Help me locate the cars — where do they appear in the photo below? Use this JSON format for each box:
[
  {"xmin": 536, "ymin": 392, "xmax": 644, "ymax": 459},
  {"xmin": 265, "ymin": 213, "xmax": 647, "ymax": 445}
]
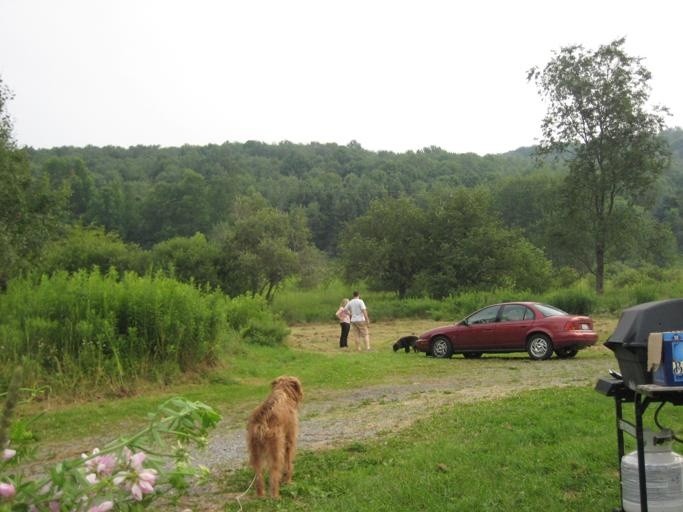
[{"xmin": 415, "ymin": 301, "xmax": 600, "ymax": 360}]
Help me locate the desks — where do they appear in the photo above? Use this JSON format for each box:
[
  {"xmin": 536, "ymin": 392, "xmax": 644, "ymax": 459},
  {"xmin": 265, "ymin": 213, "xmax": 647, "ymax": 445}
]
[{"xmin": 597, "ymin": 381, "xmax": 683, "ymax": 512}]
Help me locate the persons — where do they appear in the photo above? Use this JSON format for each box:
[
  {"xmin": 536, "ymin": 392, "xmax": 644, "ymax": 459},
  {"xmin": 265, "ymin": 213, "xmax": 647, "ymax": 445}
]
[
  {"xmin": 343, "ymin": 290, "xmax": 370, "ymax": 352},
  {"xmin": 335, "ymin": 298, "xmax": 351, "ymax": 348}
]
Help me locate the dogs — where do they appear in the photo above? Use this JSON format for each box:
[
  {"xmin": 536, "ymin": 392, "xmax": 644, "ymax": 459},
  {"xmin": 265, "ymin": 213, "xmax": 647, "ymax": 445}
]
[
  {"xmin": 246, "ymin": 375, "xmax": 304, "ymax": 501},
  {"xmin": 393, "ymin": 335, "xmax": 418, "ymax": 353}
]
